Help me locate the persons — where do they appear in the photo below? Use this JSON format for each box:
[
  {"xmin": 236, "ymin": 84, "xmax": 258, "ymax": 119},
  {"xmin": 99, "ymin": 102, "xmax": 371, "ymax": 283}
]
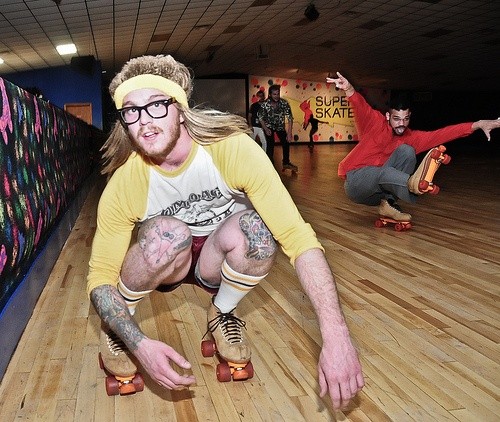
[
  {"xmin": 326, "ymin": 72, "xmax": 500, "ymax": 231},
  {"xmin": 85, "ymin": 55, "xmax": 365, "ymax": 411},
  {"xmin": 248, "ymin": 91, "xmax": 267, "ymax": 152},
  {"xmin": 258, "ymin": 85, "xmax": 298, "ymax": 173},
  {"xmin": 305, "ymin": 114, "xmax": 328, "ymax": 148}
]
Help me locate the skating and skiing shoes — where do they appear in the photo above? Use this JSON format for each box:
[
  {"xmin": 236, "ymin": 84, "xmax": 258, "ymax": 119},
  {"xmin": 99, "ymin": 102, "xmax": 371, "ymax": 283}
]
[
  {"xmin": 375, "ymin": 199, "xmax": 412, "ymax": 231},
  {"xmin": 201, "ymin": 295, "xmax": 253, "ymax": 382},
  {"xmin": 99, "ymin": 315, "xmax": 144, "ymax": 396},
  {"xmin": 408, "ymin": 145, "xmax": 451, "ymax": 196},
  {"xmin": 282, "ymin": 163, "xmax": 298, "ymax": 174}
]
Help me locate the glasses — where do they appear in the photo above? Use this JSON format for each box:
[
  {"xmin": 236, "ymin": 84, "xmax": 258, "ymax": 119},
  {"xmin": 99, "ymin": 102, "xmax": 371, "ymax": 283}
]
[{"xmin": 116, "ymin": 97, "xmax": 176, "ymax": 124}]
